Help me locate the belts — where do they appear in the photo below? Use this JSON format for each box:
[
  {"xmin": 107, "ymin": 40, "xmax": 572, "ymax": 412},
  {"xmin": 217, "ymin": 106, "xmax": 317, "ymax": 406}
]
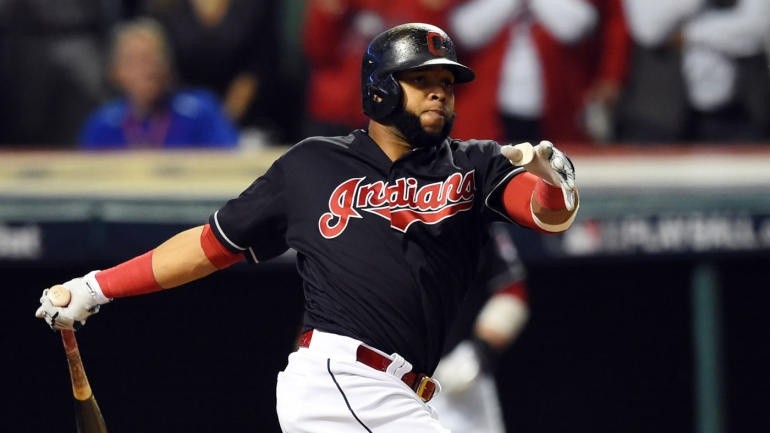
[{"xmin": 299, "ymin": 329, "xmax": 435, "ymax": 405}]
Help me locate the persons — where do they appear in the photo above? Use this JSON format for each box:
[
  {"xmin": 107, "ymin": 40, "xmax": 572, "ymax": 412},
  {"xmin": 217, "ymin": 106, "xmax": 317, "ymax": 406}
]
[
  {"xmin": 35, "ymin": 23, "xmax": 579, "ymax": 433},
  {"xmin": 69, "ymin": 16, "xmax": 238, "ymax": 149},
  {"xmin": 431, "ymin": 222, "xmax": 530, "ymax": 433},
  {"xmin": 302, "ymin": 0, "xmax": 770, "ymax": 145},
  {"xmin": 169, "ymin": 0, "xmax": 265, "ymax": 124}
]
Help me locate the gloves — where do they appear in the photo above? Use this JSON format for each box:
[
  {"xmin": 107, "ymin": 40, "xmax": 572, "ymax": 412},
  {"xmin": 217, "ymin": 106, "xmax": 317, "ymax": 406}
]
[
  {"xmin": 501, "ymin": 139, "xmax": 577, "ymax": 211},
  {"xmin": 34, "ymin": 270, "xmax": 112, "ymax": 332}
]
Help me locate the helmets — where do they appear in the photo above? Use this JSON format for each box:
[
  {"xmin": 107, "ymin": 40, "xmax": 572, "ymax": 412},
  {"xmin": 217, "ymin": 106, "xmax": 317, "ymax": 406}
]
[{"xmin": 362, "ymin": 22, "xmax": 477, "ymax": 124}]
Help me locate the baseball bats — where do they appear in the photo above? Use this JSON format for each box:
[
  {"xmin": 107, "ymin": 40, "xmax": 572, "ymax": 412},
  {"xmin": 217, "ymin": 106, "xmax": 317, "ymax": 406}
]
[{"xmin": 48, "ymin": 284, "xmax": 108, "ymax": 433}]
